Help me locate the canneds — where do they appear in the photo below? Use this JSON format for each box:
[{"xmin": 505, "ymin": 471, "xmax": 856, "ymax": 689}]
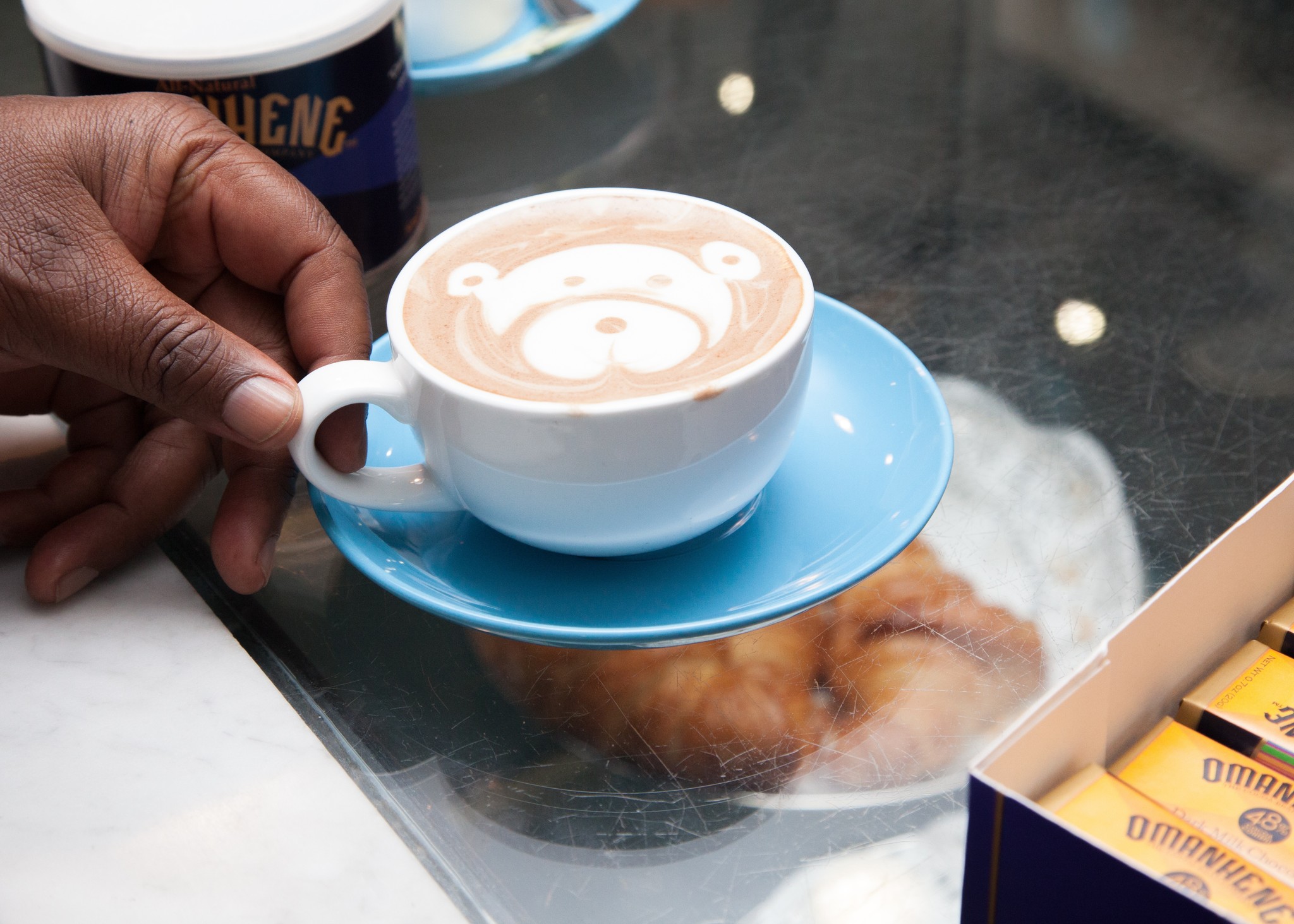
[{"xmin": 21, "ymin": 0, "xmax": 426, "ymax": 308}]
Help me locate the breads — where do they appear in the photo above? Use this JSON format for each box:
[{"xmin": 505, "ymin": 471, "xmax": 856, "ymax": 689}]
[{"xmin": 463, "ymin": 535, "xmax": 1052, "ymax": 790}]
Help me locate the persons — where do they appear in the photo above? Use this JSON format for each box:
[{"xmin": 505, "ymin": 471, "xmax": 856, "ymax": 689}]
[{"xmin": 1, "ymin": 89, "xmax": 370, "ymax": 608}]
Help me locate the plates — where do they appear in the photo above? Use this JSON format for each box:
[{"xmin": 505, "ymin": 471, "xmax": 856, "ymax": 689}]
[
  {"xmin": 382, "ymin": 1, "xmax": 643, "ymax": 91},
  {"xmin": 312, "ymin": 276, "xmax": 956, "ymax": 650},
  {"xmin": 657, "ymin": 378, "xmax": 1150, "ymax": 817}
]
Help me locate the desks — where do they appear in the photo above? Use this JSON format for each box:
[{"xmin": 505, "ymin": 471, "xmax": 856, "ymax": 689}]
[{"xmin": 5, "ymin": 1, "xmax": 1293, "ymax": 924}]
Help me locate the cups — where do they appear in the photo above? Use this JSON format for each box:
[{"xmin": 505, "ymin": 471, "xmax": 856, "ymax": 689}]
[
  {"xmin": 24, "ymin": 1, "xmax": 428, "ymax": 291},
  {"xmin": 283, "ymin": 183, "xmax": 813, "ymax": 562}
]
